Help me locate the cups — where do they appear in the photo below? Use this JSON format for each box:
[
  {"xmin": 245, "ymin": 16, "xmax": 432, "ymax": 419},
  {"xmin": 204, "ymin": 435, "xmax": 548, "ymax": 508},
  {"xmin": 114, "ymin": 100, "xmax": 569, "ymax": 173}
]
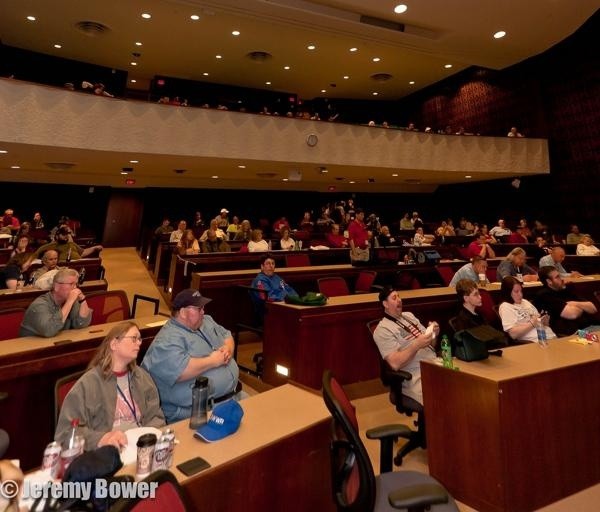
[
  {"xmin": 135, "ymin": 434, "xmax": 157, "ymax": 480},
  {"xmin": 78, "ymin": 267, "xmax": 86, "ymax": 285},
  {"xmin": 479, "ymin": 274, "xmax": 486, "ymax": 287},
  {"xmin": 65, "ymin": 248, "xmax": 74, "ymax": 262}
]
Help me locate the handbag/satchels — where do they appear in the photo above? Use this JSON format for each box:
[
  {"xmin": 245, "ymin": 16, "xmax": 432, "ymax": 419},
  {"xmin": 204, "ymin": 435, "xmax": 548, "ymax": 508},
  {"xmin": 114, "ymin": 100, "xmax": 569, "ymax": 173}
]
[
  {"xmin": 284, "ymin": 292, "xmax": 327, "ymax": 305},
  {"xmin": 454, "ymin": 324, "xmax": 504, "ymax": 362}
]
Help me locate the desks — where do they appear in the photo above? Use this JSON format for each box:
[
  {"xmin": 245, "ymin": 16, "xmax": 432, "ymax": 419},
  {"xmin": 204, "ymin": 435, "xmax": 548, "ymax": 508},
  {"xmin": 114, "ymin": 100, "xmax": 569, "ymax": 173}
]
[
  {"xmin": 1, "ymin": 278, "xmax": 108, "ymax": 317},
  {"xmin": 164, "ymin": 242, "xmax": 600, "ymax": 301},
  {"xmin": 189, "ymin": 256, "xmax": 599, "ymax": 336},
  {"xmin": 420, "ymin": 330, "xmax": 600, "ymax": 511},
  {"xmin": 2, "ymin": 257, "xmax": 104, "ymax": 289},
  {"xmin": 0, "ymin": 235, "xmax": 95, "ymax": 250},
  {"xmin": 152, "ymin": 239, "xmax": 295, "ymax": 285},
  {"xmin": 16, "ymin": 380, "xmax": 357, "ymax": 511},
  {"xmin": 261, "ymin": 274, "xmax": 600, "ymax": 390},
  {"xmin": 1, "ymin": 314, "xmax": 175, "ymax": 443}
]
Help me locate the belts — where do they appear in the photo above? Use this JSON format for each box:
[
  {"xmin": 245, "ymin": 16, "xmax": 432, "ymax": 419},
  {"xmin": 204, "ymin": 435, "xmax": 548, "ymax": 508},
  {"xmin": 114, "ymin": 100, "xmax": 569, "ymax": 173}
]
[{"xmin": 208, "ymin": 381, "xmax": 242, "ymax": 408}]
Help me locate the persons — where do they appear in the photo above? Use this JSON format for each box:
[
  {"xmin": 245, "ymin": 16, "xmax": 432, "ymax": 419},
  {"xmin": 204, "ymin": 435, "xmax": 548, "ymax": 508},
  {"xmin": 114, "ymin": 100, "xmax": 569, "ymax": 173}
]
[
  {"xmin": 534, "ymin": 266, "xmax": 600, "ymax": 335},
  {"xmin": 19, "ymin": 269, "xmax": 95, "ymax": 378},
  {"xmin": 155, "ymin": 199, "xmax": 600, "ymax": 262},
  {"xmin": 0, "ymin": 208, "xmax": 106, "ymax": 290},
  {"xmin": 448, "ymin": 255, "xmax": 490, "ymax": 288},
  {"xmin": 250, "ymin": 254, "xmax": 299, "ymax": 304},
  {"xmin": 373, "ymin": 286, "xmax": 444, "ymax": 407},
  {"xmin": 538, "ymin": 247, "xmax": 583, "ymax": 278},
  {"xmin": 63, "ymin": 82, "xmax": 522, "ymax": 137},
  {"xmin": 497, "ymin": 276, "xmax": 558, "ymax": 343},
  {"xmin": 53, "ymin": 321, "xmax": 167, "ymax": 454},
  {"xmin": 496, "ymin": 247, "xmax": 539, "ymax": 281},
  {"xmin": 139, "ymin": 288, "xmax": 251, "ymax": 426},
  {"xmin": 454, "ymin": 278, "xmax": 506, "ymax": 350}
]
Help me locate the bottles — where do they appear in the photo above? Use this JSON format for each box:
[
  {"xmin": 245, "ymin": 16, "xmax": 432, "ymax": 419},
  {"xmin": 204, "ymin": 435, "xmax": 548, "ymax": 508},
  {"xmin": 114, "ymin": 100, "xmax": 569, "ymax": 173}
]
[
  {"xmin": 190, "ymin": 376, "xmax": 209, "ymax": 429},
  {"xmin": 58, "ymin": 418, "xmax": 85, "ymax": 480},
  {"xmin": 23, "ymin": 272, "xmax": 36, "ymax": 288},
  {"xmin": 535, "ymin": 318, "xmax": 550, "ymax": 349},
  {"xmin": 515, "ymin": 265, "xmax": 523, "ymax": 285},
  {"xmin": 441, "ymin": 334, "xmax": 453, "ymax": 368},
  {"xmin": 16, "ymin": 270, "xmax": 25, "ymax": 290}
]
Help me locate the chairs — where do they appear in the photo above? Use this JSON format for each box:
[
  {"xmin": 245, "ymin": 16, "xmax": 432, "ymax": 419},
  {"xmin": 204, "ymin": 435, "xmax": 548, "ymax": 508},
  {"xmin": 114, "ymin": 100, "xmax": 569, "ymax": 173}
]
[
  {"xmin": 448, "ymin": 316, "xmax": 458, "ymax": 333},
  {"xmin": 492, "ymin": 306, "xmax": 499, "ymax": 319},
  {"xmin": 322, "ymin": 369, "xmax": 462, "ymax": 511},
  {"xmin": 80, "ymin": 288, "xmax": 129, "ymax": 325},
  {"xmin": 367, "ymin": 320, "xmax": 425, "ymax": 466},
  {"xmin": 394, "ymin": 268, "xmax": 441, "ymax": 290},
  {"xmin": 432, "ymin": 263, "xmax": 464, "ymax": 287},
  {"xmin": 352, "ymin": 270, "xmax": 385, "ymax": 292},
  {"xmin": 456, "ymin": 246, "xmax": 470, "ymax": 260},
  {"xmin": 375, "ymin": 248, "xmax": 400, "ymax": 260},
  {"xmin": 317, "ymin": 277, "xmax": 350, "ymax": 296},
  {"xmin": 230, "ymin": 279, "xmax": 270, "ymax": 376},
  {"xmin": 54, "ymin": 369, "xmax": 85, "ymax": 432},
  {"xmin": 293, "ymin": 231, "xmax": 312, "ymax": 246},
  {"xmin": 285, "ymin": 253, "xmax": 311, "ymax": 266},
  {"xmin": 485, "ymin": 267, "xmax": 498, "ymax": 283},
  {"xmin": 0, "ymin": 307, "xmax": 26, "ymax": 342}
]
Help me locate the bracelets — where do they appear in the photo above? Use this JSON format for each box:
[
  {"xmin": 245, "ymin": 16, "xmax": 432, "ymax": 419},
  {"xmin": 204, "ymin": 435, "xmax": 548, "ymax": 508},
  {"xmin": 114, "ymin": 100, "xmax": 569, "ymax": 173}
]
[
  {"xmin": 529, "ymin": 320, "xmax": 536, "ymax": 329},
  {"xmin": 78, "ymin": 296, "xmax": 86, "ymax": 305}
]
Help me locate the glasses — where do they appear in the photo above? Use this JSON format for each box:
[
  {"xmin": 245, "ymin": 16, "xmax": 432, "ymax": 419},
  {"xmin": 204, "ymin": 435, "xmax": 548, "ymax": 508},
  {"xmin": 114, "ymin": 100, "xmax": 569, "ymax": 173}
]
[
  {"xmin": 58, "ymin": 282, "xmax": 79, "ymax": 288},
  {"xmin": 121, "ymin": 335, "xmax": 142, "ymax": 345},
  {"xmin": 187, "ymin": 306, "xmax": 204, "ymax": 311}
]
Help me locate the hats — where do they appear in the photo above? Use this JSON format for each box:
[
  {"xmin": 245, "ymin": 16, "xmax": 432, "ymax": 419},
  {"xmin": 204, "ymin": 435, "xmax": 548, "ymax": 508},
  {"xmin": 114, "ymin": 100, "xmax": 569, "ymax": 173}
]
[
  {"xmin": 174, "ymin": 289, "xmax": 212, "ymax": 306},
  {"xmin": 193, "ymin": 399, "xmax": 244, "ymax": 443},
  {"xmin": 378, "ymin": 286, "xmax": 395, "ymax": 301},
  {"xmin": 221, "ymin": 208, "xmax": 229, "ymax": 213},
  {"xmin": 55, "ymin": 227, "xmax": 71, "ymax": 235}
]
